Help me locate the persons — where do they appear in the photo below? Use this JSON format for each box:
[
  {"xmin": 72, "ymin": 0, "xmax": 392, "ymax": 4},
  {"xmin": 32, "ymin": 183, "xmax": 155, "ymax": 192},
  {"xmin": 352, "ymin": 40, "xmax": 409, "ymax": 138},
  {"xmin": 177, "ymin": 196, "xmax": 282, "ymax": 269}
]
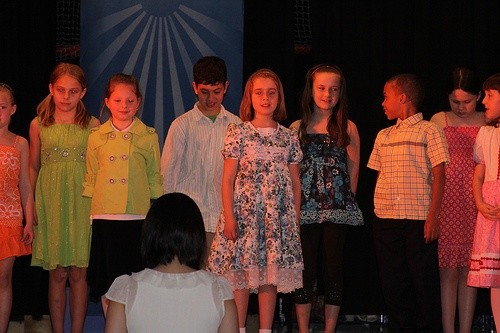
[
  {"xmin": 207, "ymin": 70, "xmax": 304, "ymax": 333},
  {"xmin": 466, "ymin": 72, "xmax": 500, "ymax": 333},
  {"xmin": 0, "ymin": 82, "xmax": 34, "ymax": 333},
  {"xmin": 82, "ymin": 74, "xmax": 164, "ymax": 301},
  {"xmin": 289, "ymin": 65, "xmax": 364, "ymax": 333},
  {"xmin": 429, "ymin": 65, "xmax": 486, "ymax": 333},
  {"xmin": 160, "ymin": 56, "xmax": 243, "ymax": 252},
  {"xmin": 101, "ymin": 191, "xmax": 240, "ymax": 333},
  {"xmin": 366, "ymin": 73, "xmax": 444, "ymax": 333},
  {"xmin": 30, "ymin": 63, "xmax": 100, "ymax": 333}
]
